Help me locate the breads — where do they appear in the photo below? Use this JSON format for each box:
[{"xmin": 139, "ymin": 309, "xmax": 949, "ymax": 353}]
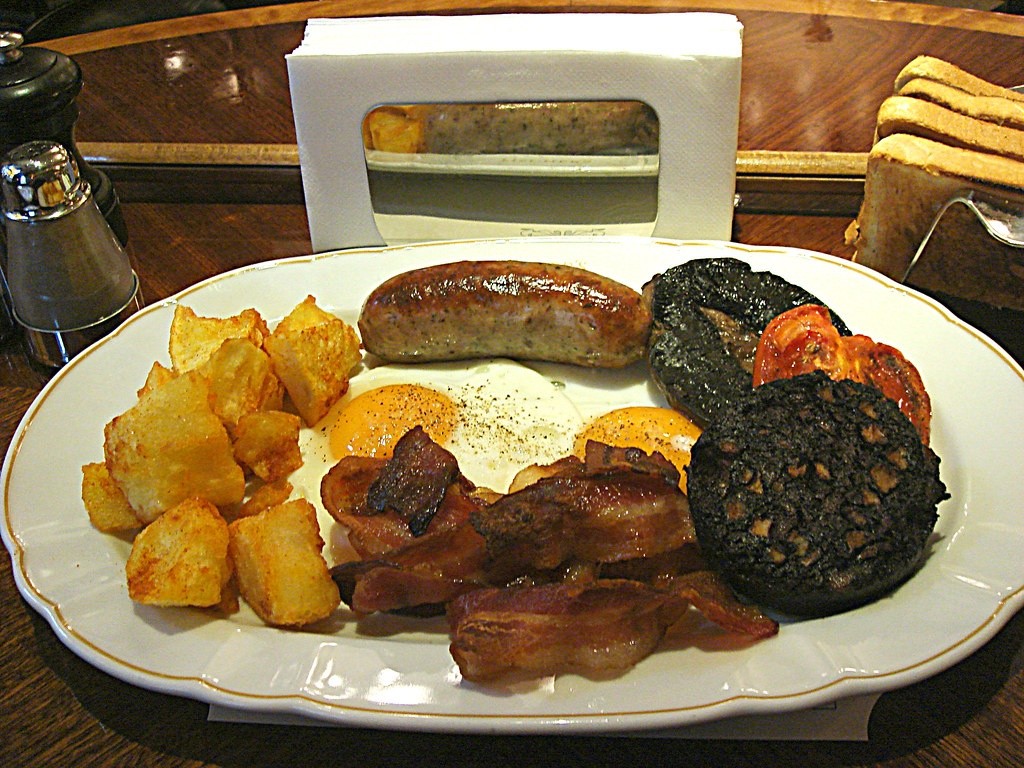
[{"xmin": 845, "ymin": 55, "xmax": 1024, "ymax": 308}]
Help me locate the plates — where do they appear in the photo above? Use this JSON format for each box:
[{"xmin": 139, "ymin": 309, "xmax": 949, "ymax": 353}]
[{"xmin": 0, "ymin": 234, "xmax": 1024, "ymax": 733}]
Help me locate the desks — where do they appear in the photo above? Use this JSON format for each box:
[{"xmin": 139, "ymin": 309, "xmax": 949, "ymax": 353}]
[{"xmin": 2, "ymin": 0, "xmax": 1024, "ymax": 768}]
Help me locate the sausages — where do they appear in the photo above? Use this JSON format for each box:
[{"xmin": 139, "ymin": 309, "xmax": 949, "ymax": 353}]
[{"xmin": 357, "ymin": 261, "xmax": 649, "ymax": 370}]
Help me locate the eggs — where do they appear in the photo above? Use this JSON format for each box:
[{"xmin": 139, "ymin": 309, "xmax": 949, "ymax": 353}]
[{"xmin": 287, "ymin": 356, "xmax": 701, "ymax": 569}]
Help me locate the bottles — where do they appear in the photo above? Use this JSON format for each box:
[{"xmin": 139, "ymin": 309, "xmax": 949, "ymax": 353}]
[{"xmin": 0, "ymin": 140, "xmax": 143, "ymax": 368}]
